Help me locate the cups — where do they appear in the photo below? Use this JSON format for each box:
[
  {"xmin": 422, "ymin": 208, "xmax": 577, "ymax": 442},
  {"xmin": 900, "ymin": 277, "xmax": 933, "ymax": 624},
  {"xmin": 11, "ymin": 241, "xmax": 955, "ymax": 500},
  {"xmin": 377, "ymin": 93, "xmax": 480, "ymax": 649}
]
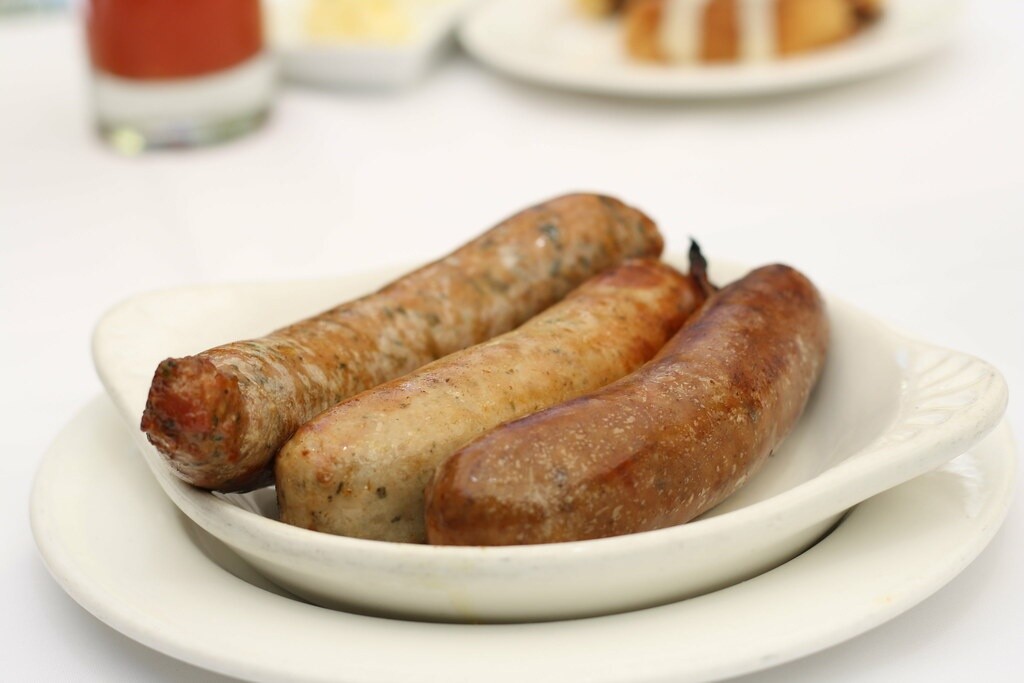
[{"xmin": 73, "ymin": 0, "xmax": 277, "ymax": 149}]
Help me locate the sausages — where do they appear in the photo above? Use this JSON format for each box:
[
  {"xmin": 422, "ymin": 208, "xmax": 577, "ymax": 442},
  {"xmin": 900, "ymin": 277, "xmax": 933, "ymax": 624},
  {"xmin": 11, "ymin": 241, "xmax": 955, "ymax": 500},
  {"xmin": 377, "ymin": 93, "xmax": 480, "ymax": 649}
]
[{"xmin": 139, "ymin": 194, "xmax": 831, "ymax": 548}]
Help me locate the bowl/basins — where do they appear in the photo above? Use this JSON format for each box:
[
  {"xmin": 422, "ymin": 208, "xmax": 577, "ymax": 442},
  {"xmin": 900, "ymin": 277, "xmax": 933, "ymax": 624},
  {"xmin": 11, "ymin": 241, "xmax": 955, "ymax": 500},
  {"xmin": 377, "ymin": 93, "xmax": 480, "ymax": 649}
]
[{"xmin": 93, "ymin": 283, "xmax": 1007, "ymax": 622}]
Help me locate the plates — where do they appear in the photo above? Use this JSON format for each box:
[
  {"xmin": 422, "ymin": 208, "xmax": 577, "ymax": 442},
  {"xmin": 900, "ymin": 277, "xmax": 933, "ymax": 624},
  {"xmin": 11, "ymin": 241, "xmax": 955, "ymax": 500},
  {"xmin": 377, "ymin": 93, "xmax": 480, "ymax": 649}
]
[
  {"xmin": 28, "ymin": 389, "xmax": 1014, "ymax": 682},
  {"xmin": 454, "ymin": 0, "xmax": 959, "ymax": 98}
]
